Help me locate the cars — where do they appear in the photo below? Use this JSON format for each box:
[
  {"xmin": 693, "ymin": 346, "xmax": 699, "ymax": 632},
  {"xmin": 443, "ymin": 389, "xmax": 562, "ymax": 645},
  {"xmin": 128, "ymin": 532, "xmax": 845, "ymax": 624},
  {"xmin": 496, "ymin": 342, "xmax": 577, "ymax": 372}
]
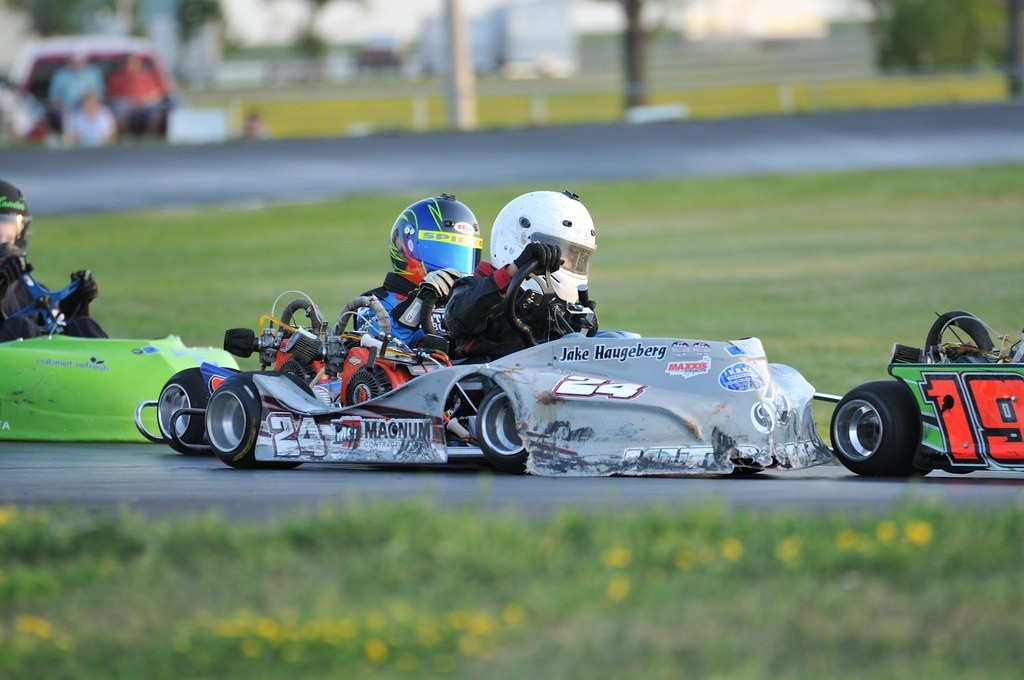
[{"xmin": 10, "ymin": 39, "xmax": 187, "ymax": 146}]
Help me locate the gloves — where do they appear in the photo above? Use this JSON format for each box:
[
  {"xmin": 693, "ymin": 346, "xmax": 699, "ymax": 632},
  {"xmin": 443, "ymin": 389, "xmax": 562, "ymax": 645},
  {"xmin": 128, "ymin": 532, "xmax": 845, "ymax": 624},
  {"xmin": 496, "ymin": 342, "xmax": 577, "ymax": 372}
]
[
  {"xmin": 68, "ymin": 269, "xmax": 99, "ymax": 306},
  {"xmin": 514, "ymin": 242, "xmax": 565, "ymax": 281},
  {"xmin": 0, "ymin": 251, "xmax": 33, "ymax": 298},
  {"xmin": 390, "ymin": 268, "xmax": 460, "ymax": 330}
]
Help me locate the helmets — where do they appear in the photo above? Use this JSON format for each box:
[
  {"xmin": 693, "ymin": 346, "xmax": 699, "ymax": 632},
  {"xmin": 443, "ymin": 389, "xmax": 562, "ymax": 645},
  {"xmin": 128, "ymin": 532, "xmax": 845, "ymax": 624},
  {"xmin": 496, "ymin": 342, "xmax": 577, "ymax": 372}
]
[
  {"xmin": 389, "ymin": 193, "xmax": 484, "ymax": 285},
  {"xmin": 0, "ymin": 180, "xmax": 34, "ymax": 256},
  {"xmin": 489, "ymin": 190, "xmax": 598, "ymax": 303}
]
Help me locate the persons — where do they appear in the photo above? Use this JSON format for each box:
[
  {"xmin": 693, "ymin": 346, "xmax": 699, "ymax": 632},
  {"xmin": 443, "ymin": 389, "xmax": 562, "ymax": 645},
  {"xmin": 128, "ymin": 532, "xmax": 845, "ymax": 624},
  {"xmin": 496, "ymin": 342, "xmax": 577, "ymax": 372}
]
[
  {"xmin": 61, "ymin": 95, "xmax": 118, "ymax": 147},
  {"xmin": 50, "ymin": 55, "xmax": 105, "ymax": 116},
  {"xmin": 248, "ymin": 114, "xmax": 264, "ymax": 139},
  {"xmin": 107, "ymin": 54, "xmax": 173, "ymax": 139},
  {"xmin": 356, "ymin": 193, "xmax": 484, "ymax": 347},
  {"xmin": 0, "ymin": 75, "xmax": 59, "ymax": 150},
  {"xmin": 0, "ymin": 180, "xmax": 108, "ymax": 342},
  {"xmin": 443, "ymin": 190, "xmax": 599, "ymax": 362}
]
